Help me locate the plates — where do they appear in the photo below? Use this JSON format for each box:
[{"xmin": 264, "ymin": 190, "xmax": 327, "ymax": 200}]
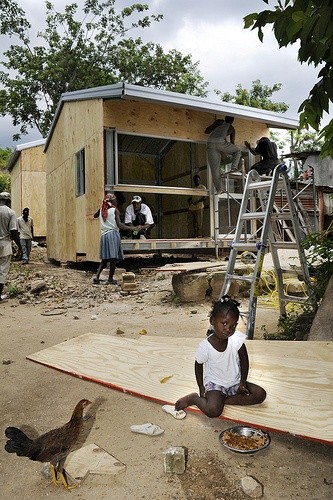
[{"xmin": 219, "ymin": 426, "xmax": 271, "ymax": 454}]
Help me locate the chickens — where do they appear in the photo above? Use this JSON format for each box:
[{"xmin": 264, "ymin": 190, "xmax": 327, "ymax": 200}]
[{"xmin": 4, "ymin": 399, "xmax": 92, "ymax": 493}]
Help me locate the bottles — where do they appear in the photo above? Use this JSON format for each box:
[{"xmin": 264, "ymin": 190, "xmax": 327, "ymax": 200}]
[{"xmin": 133, "ymin": 230, "xmax": 139, "ymax": 235}]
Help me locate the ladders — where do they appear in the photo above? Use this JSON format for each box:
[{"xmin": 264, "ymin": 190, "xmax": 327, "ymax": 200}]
[{"xmin": 207, "ymin": 154, "xmax": 319, "ymax": 340}]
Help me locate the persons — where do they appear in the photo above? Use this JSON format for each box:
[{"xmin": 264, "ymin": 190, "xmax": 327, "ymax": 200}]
[
  {"xmin": 187, "ymin": 174, "xmax": 207, "ymax": 238},
  {"xmin": 0, "ymin": 192, "xmax": 24, "ymax": 304},
  {"xmin": 299, "ymin": 164, "xmax": 315, "ymax": 181},
  {"xmin": 92, "ymin": 193, "xmax": 138, "ymax": 285},
  {"xmin": 16, "ymin": 207, "xmax": 34, "ymax": 264},
  {"xmin": 204, "ymin": 116, "xmax": 242, "ymax": 195},
  {"xmin": 122, "ymin": 195, "xmax": 154, "ymax": 240},
  {"xmin": 174, "ymin": 294, "xmax": 267, "ymax": 418},
  {"xmin": 244, "ymin": 136, "xmax": 281, "ymax": 177}
]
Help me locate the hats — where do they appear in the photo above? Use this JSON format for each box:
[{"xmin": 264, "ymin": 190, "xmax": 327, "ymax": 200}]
[
  {"xmin": 131, "ymin": 195, "xmax": 142, "ymax": 203},
  {"xmin": 0, "ymin": 191, "xmax": 12, "ymax": 203}
]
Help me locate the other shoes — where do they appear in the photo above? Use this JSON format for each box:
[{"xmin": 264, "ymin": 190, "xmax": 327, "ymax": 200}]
[
  {"xmin": 107, "ymin": 279, "xmax": 118, "ymax": 284},
  {"xmin": 196, "ymin": 234, "xmax": 204, "ymax": 238},
  {"xmin": 21, "ymin": 259, "xmax": 29, "ymax": 265},
  {"xmin": 217, "ymin": 191, "xmax": 223, "ymax": 194},
  {"xmin": 139, "ymin": 235, "xmax": 146, "ymax": 239},
  {"xmin": 91, "ymin": 278, "xmax": 101, "ymax": 284},
  {"xmin": 230, "ymin": 168, "xmax": 238, "ymax": 173}
]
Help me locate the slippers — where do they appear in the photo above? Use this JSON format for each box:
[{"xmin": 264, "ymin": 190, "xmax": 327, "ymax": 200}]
[
  {"xmin": 162, "ymin": 404, "xmax": 186, "ymax": 420},
  {"xmin": 130, "ymin": 421, "xmax": 165, "ymax": 436}
]
